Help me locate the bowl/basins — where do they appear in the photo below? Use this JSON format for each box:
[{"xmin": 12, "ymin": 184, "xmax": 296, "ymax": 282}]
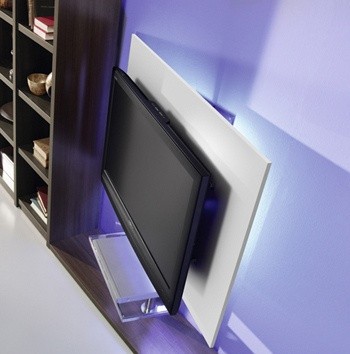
[
  {"xmin": 45, "ymin": 71, "xmax": 52, "ymax": 98},
  {"xmin": 26, "ymin": 73, "xmax": 49, "ymax": 96}
]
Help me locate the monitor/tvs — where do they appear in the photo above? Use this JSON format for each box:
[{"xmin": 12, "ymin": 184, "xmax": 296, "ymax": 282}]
[{"xmin": 100, "ymin": 66, "xmax": 210, "ymax": 315}]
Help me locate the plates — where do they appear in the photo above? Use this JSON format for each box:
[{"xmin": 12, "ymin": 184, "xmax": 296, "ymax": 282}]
[{"xmin": 0, "ymin": 101, "xmax": 13, "ymax": 123}]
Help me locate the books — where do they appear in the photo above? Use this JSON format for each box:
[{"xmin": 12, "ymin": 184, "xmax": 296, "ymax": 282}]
[
  {"xmin": 33, "ymin": 14, "xmax": 54, "ymax": 33},
  {"xmin": 33, "ymin": 25, "xmax": 54, "ymax": 41},
  {"xmin": 32, "ymin": 148, "xmax": 49, "ymax": 170},
  {"xmin": 29, "ymin": 186, "xmax": 48, "ymax": 225},
  {"xmin": 33, "ymin": 137, "xmax": 50, "ymax": 161}
]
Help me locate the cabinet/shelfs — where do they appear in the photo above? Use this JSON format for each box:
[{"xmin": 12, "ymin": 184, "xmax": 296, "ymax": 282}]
[{"xmin": 0, "ymin": 0, "xmax": 123, "ymax": 248}]
